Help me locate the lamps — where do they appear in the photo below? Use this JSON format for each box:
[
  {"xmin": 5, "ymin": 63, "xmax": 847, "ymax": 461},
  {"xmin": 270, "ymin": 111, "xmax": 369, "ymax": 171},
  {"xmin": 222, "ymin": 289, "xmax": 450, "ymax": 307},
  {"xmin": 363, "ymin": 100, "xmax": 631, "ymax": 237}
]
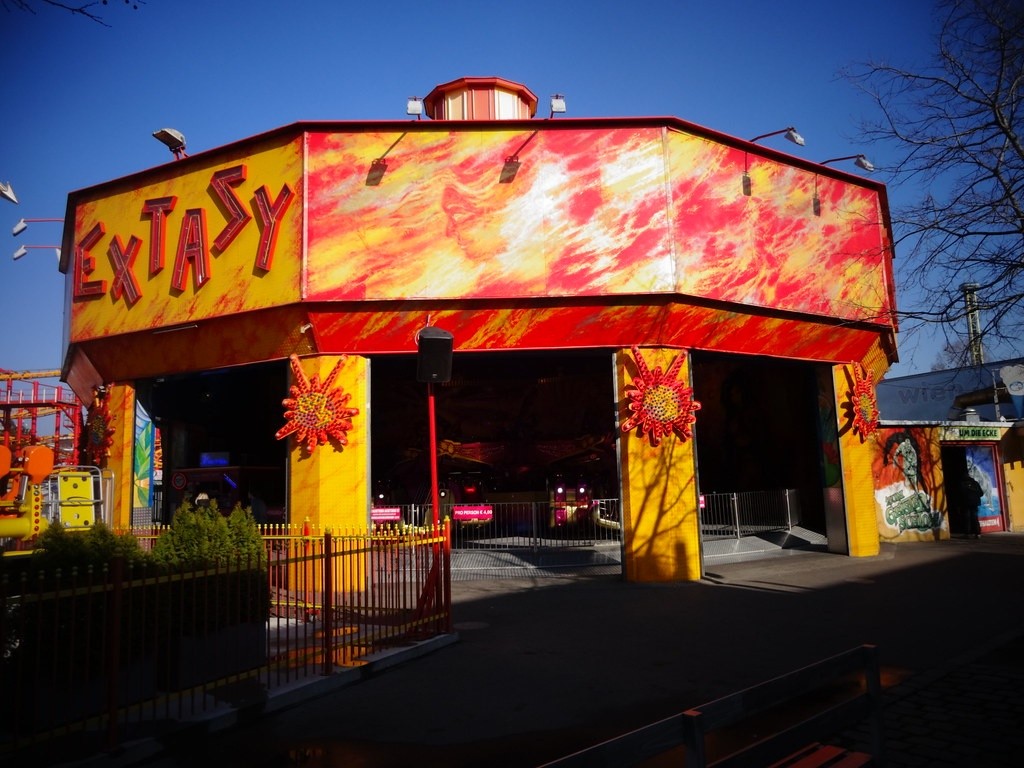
[
  {"xmin": 407, "ymin": 96, "xmax": 424, "ymax": 120},
  {"xmin": 820, "ymin": 155, "xmax": 874, "ymax": 171},
  {"xmin": 13, "ymin": 245, "xmax": 61, "ymax": 260},
  {"xmin": 152, "ymin": 128, "xmax": 190, "ymax": 160},
  {"xmin": 13, "ymin": 218, "xmax": 65, "ymax": 237},
  {"xmin": 549, "ymin": 95, "xmax": 566, "ymax": 118},
  {"xmin": 749, "ymin": 126, "xmax": 804, "ymax": 146}
]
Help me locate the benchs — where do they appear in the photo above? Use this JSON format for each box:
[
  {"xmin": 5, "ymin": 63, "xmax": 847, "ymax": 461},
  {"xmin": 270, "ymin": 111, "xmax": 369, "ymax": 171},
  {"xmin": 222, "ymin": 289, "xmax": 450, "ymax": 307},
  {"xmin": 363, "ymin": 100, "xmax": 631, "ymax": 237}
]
[{"xmin": 536, "ymin": 644, "xmax": 886, "ymax": 768}]
